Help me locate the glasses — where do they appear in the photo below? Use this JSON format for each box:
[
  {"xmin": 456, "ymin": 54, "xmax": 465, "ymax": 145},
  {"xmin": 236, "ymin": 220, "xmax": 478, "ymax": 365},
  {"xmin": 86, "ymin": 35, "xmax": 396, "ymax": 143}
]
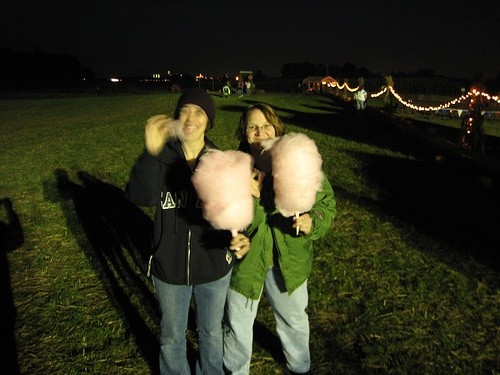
[{"xmin": 246, "ymin": 123, "xmax": 272, "ymax": 131}]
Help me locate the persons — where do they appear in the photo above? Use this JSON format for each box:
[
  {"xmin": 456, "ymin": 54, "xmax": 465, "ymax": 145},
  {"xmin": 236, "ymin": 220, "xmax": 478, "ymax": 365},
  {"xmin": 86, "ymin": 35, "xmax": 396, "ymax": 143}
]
[
  {"xmin": 222, "ymin": 102, "xmax": 338, "ymax": 375},
  {"xmin": 125, "ymin": 86, "xmax": 257, "ymax": 375},
  {"xmin": 353, "ymin": 85, "xmax": 368, "ymax": 110},
  {"xmin": 408, "ymin": 141, "xmax": 500, "ymax": 272}
]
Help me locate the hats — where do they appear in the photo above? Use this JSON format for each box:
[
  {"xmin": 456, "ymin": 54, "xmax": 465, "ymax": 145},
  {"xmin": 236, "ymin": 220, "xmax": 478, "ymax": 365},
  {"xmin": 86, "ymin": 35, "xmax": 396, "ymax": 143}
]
[{"xmin": 175, "ymin": 88, "xmax": 215, "ymax": 131}]
